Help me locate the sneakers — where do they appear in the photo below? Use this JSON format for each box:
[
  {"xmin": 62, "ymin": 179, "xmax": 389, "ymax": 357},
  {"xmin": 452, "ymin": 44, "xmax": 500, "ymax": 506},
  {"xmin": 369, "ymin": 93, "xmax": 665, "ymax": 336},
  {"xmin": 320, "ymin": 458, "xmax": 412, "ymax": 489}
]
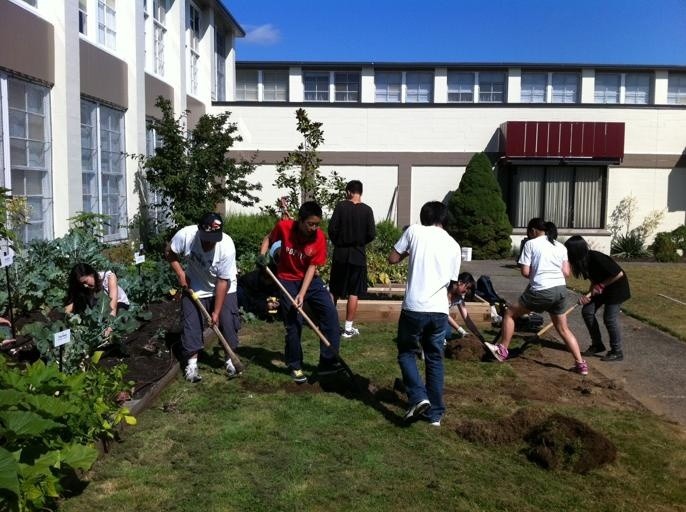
[
  {"xmin": 289, "ymin": 361, "xmax": 307, "ymax": 381},
  {"xmin": 600, "ymin": 351, "xmax": 624, "ymax": 361},
  {"xmin": 484, "ymin": 342, "xmax": 509, "ymax": 362},
  {"xmin": 576, "ymin": 360, "xmax": 589, "ymax": 375},
  {"xmin": 581, "ymin": 344, "xmax": 606, "ymax": 355},
  {"xmin": 403, "ymin": 399, "xmax": 441, "ymax": 426},
  {"xmin": 317, "ymin": 364, "xmax": 345, "ymax": 375},
  {"xmin": 341, "ymin": 328, "xmax": 360, "ymax": 338},
  {"xmin": 185, "ymin": 365, "xmax": 202, "ymax": 383},
  {"xmin": 225, "ymin": 358, "xmax": 242, "ymax": 377}
]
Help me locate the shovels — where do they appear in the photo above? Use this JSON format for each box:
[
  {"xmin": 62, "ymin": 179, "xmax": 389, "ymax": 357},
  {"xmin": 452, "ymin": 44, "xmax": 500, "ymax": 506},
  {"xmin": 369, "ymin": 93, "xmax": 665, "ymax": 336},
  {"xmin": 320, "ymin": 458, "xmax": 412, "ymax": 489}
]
[
  {"xmin": 265, "ymin": 266, "xmax": 379, "ymax": 395},
  {"xmin": 508, "ymin": 292, "xmax": 592, "ymax": 356}
]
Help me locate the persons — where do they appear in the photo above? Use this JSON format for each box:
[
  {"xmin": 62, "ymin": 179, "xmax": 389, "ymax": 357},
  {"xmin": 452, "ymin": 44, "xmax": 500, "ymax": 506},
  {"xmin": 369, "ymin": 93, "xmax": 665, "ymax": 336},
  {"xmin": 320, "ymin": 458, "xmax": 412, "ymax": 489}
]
[
  {"xmin": 255, "ymin": 201, "xmax": 346, "ymax": 383},
  {"xmin": 484, "ymin": 218, "xmax": 588, "ymax": 375},
  {"xmin": 164, "ymin": 212, "xmax": 241, "ymax": 383},
  {"xmin": 237, "ymin": 264, "xmax": 284, "ymax": 315},
  {"xmin": 64, "ymin": 263, "xmax": 130, "ymax": 336},
  {"xmin": 565, "ymin": 236, "xmax": 631, "ymax": 362},
  {"xmin": 419, "ymin": 272, "xmax": 486, "ymax": 360},
  {"xmin": 328, "ymin": 181, "xmax": 375, "ymax": 338},
  {"xmin": 389, "ymin": 202, "xmax": 463, "ymax": 427}
]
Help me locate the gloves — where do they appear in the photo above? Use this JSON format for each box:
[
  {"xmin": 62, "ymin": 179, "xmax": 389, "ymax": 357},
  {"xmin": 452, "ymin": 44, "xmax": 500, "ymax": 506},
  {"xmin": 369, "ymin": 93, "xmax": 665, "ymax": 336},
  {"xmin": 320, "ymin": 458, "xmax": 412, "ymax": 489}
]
[
  {"xmin": 577, "ymin": 296, "xmax": 591, "ymax": 305},
  {"xmin": 593, "ymin": 283, "xmax": 605, "ymax": 296},
  {"xmin": 457, "ymin": 326, "xmax": 469, "ymax": 338}
]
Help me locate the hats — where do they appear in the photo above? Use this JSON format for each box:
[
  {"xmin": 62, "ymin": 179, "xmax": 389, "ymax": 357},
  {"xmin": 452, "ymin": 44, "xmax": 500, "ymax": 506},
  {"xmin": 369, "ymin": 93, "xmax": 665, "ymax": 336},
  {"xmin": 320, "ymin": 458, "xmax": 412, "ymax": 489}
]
[{"xmin": 198, "ymin": 212, "xmax": 223, "ymax": 242}]
